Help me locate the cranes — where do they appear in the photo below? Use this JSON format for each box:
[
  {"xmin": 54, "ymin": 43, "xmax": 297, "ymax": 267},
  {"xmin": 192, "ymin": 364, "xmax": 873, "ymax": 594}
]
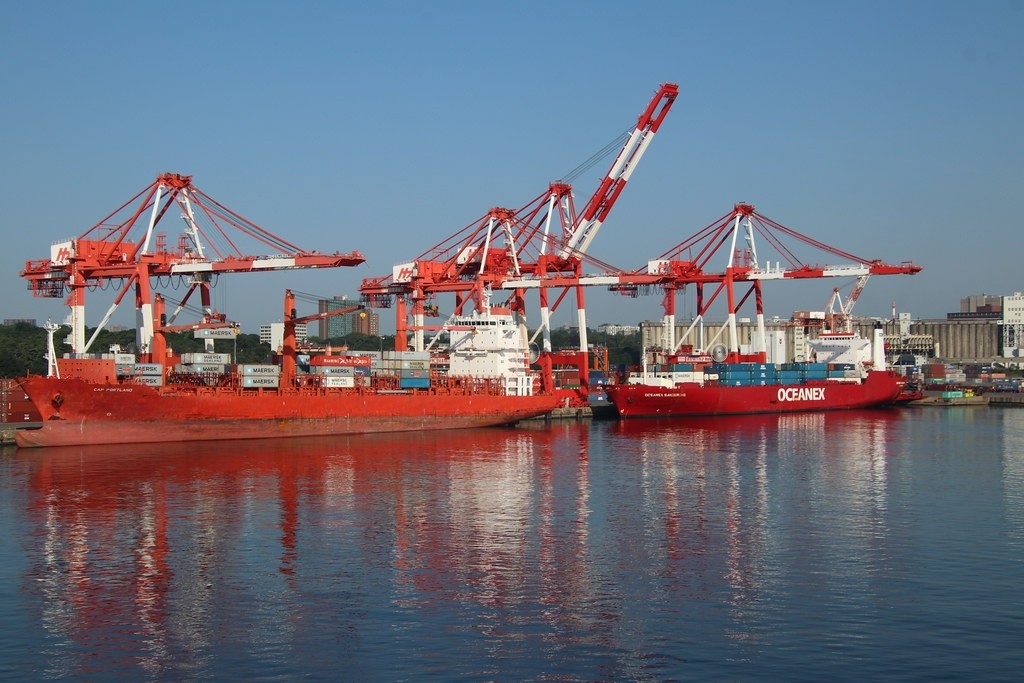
[{"xmin": 27, "ymin": 81, "xmax": 920, "ymax": 361}]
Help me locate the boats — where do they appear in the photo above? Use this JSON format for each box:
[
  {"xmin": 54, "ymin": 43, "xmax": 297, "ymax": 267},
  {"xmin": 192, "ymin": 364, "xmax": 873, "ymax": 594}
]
[
  {"xmin": 599, "ymin": 319, "xmax": 924, "ymax": 419},
  {"xmin": 11, "ymin": 286, "xmax": 561, "ymax": 451}
]
